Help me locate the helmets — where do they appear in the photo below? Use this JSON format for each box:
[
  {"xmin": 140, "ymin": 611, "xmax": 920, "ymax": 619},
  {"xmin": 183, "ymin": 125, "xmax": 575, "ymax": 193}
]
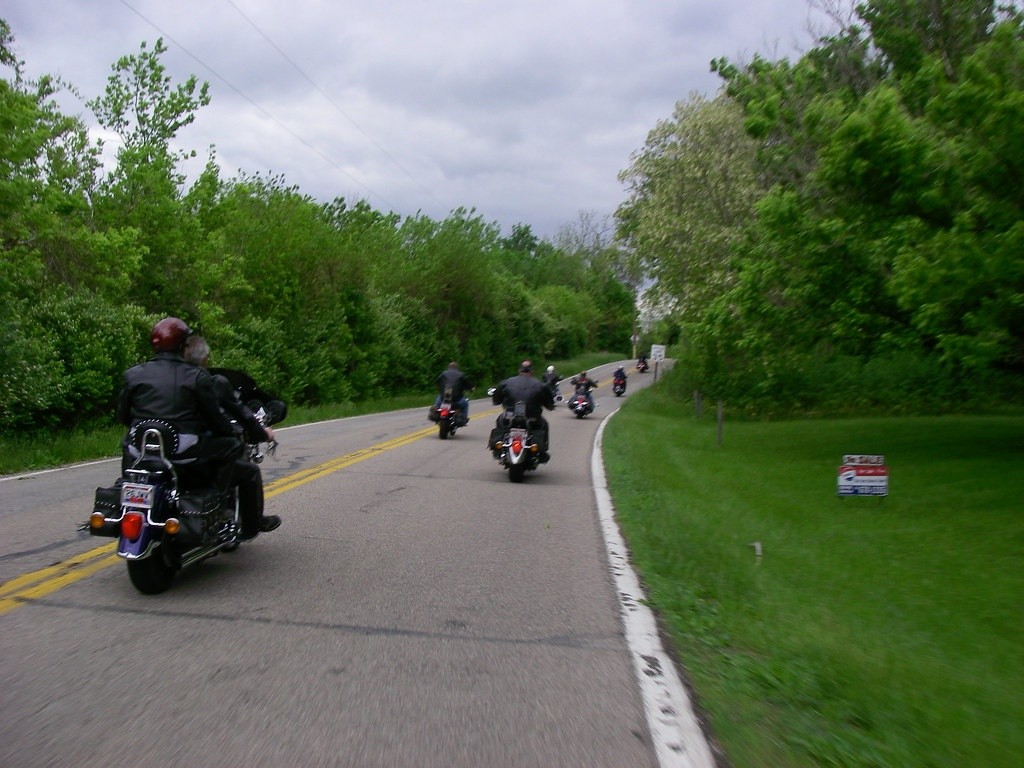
[
  {"xmin": 547, "ymin": 366, "xmax": 555, "ymax": 373},
  {"xmin": 619, "ymin": 365, "xmax": 624, "ymax": 370},
  {"xmin": 151, "ymin": 317, "xmax": 190, "ymax": 349}
]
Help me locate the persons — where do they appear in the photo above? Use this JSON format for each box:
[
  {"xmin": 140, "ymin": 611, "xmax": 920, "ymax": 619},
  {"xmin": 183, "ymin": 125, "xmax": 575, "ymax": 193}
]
[
  {"xmin": 433, "ymin": 361, "xmax": 475, "ymax": 428},
  {"xmin": 110, "ymin": 317, "xmax": 281, "ymax": 544},
  {"xmin": 636, "ymin": 352, "xmax": 650, "ymax": 370},
  {"xmin": 489, "ymin": 361, "xmax": 550, "ymax": 452},
  {"xmin": 543, "ymin": 365, "xmax": 563, "ymax": 398},
  {"xmin": 568, "ymin": 370, "xmax": 597, "ymax": 408},
  {"xmin": 612, "ymin": 365, "xmax": 628, "ymax": 393}
]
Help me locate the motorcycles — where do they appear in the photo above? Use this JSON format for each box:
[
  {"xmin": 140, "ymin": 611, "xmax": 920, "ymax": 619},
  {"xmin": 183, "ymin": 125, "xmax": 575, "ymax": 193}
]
[
  {"xmin": 637, "ymin": 363, "xmax": 648, "ymax": 373},
  {"xmin": 567, "ymin": 377, "xmax": 599, "ymax": 420},
  {"xmin": 551, "ymin": 375, "xmax": 565, "ymax": 407},
  {"xmin": 612, "ymin": 374, "xmax": 631, "ymax": 397},
  {"xmin": 88, "ymin": 368, "xmax": 291, "ymax": 595},
  {"xmin": 485, "ymin": 386, "xmax": 565, "ymax": 483},
  {"xmin": 428, "ymin": 383, "xmax": 477, "ymax": 441}
]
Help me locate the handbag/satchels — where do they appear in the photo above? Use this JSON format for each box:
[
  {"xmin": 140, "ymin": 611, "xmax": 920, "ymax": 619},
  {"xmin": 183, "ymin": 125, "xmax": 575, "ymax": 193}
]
[
  {"xmin": 87, "ymin": 487, "xmax": 121, "ymax": 539},
  {"xmin": 178, "ymin": 493, "xmax": 222, "ymax": 541}
]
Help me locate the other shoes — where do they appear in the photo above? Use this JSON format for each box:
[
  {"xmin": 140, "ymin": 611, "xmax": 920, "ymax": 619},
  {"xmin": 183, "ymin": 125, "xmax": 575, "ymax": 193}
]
[{"xmin": 242, "ymin": 515, "xmax": 282, "ymax": 536}]
